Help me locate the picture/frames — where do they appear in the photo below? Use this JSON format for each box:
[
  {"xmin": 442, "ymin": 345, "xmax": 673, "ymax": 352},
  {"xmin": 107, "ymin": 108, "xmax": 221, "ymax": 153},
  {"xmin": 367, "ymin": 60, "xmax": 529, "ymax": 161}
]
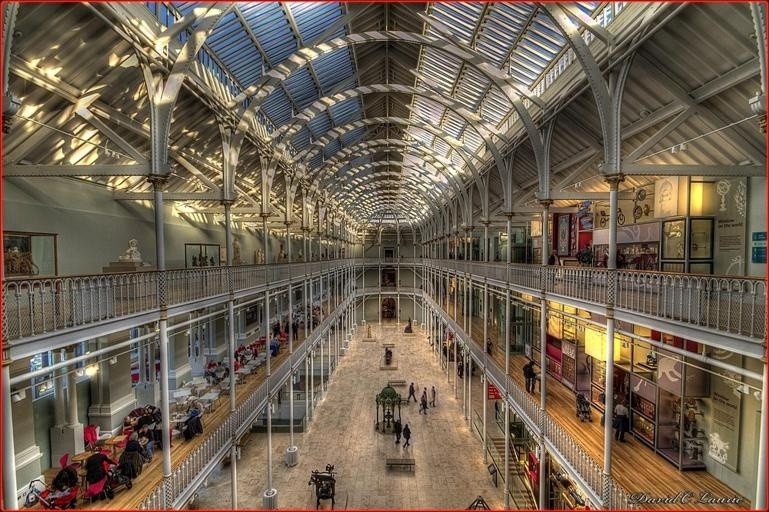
[
  {"xmin": 173, "ymin": 353, "xmax": 266, "ymax": 438},
  {"xmin": 106, "ymin": 434, "xmax": 125, "ymax": 458},
  {"xmin": 72, "ymin": 450, "xmax": 100, "ymax": 470}
]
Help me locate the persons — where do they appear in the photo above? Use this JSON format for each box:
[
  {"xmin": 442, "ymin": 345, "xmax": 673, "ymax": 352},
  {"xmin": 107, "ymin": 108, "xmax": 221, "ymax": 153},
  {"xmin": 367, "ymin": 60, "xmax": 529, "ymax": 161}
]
[
  {"xmin": 614, "ymin": 399, "xmax": 630, "ymax": 442},
  {"xmin": 283, "ymin": 247, "xmax": 288, "ymax": 258},
  {"xmin": 52, "ymin": 399, "xmax": 205, "ymax": 508},
  {"xmin": 419, "ymin": 395, "xmax": 427, "ymax": 414},
  {"xmin": 420, "ymin": 387, "xmax": 429, "ymax": 409},
  {"xmin": 403, "ymin": 424, "xmax": 411, "ymax": 447},
  {"xmin": 232, "ymin": 237, "xmax": 240, "ymax": 260},
  {"xmin": 429, "ymin": 386, "xmax": 436, "ymax": 407},
  {"xmin": 394, "ymin": 420, "xmax": 402, "ymax": 443},
  {"xmin": 256, "ymin": 248, "xmax": 261, "ymax": 264},
  {"xmin": 277, "ymin": 250, "xmax": 284, "ymax": 262},
  {"xmin": 523, "ymin": 361, "xmax": 535, "ymax": 393},
  {"xmin": 548, "ymin": 250, "xmax": 560, "ymax": 285},
  {"xmin": 459, "ymin": 362, "xmax": 464, "ymax": 378},
  {"xmin": 408, "ymin": 382, "xmax": 417, "ymax": 403},
  {"xmin": 296, "ymin": 249, "xmax": 302, "ymax": 261},
  {"xmin": 207, "ymin": 304, "xmax": 325, "ymax": 381},
  {"xmin": 261, "ymin": 249, "xmax": 265, "ymax": 260},
  {"xmin": 487, "ymin": 337, "xmax": 493, "ymax": 356}
]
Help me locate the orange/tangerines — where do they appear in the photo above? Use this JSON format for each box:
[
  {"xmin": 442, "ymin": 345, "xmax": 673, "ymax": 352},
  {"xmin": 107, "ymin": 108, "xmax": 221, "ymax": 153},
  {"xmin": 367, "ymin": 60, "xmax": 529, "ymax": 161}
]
[
  {"xmin": 28, "ymin": 468, "xmax": 80, "ymax": 511},
  {"xmin": 98, "ymin": 463, "xmax": 132, "ymax": 500},
  {"xmin": 574, "ymin": 392, "xmax": 592, "ymax": 423}
]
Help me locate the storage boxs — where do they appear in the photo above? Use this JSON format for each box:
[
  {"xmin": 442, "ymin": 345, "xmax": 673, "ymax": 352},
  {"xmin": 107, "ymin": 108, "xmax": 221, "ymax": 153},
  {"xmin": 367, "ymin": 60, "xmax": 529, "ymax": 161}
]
[
  {"xmin": 388, "ymin": 380, "xmax": 406, "ymax": 385},
  {"xmin": 386, "ymin": 459, "xmax": 415, "ymax": 470}
]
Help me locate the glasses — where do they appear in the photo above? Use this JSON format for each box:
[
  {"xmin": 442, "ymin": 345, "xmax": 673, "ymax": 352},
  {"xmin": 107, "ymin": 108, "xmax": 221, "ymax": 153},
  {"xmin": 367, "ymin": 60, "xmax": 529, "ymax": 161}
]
[
  {"xmin": 524, "ymin": 114, "xmax": 758, "ymax": 206},
  {"xmin": 11, "ymin": 287, "xmax": 303, "ymax": 403},
  {"xmin": 448, "ymin": 279, "xmax": 762, "ymax": 400}
]
